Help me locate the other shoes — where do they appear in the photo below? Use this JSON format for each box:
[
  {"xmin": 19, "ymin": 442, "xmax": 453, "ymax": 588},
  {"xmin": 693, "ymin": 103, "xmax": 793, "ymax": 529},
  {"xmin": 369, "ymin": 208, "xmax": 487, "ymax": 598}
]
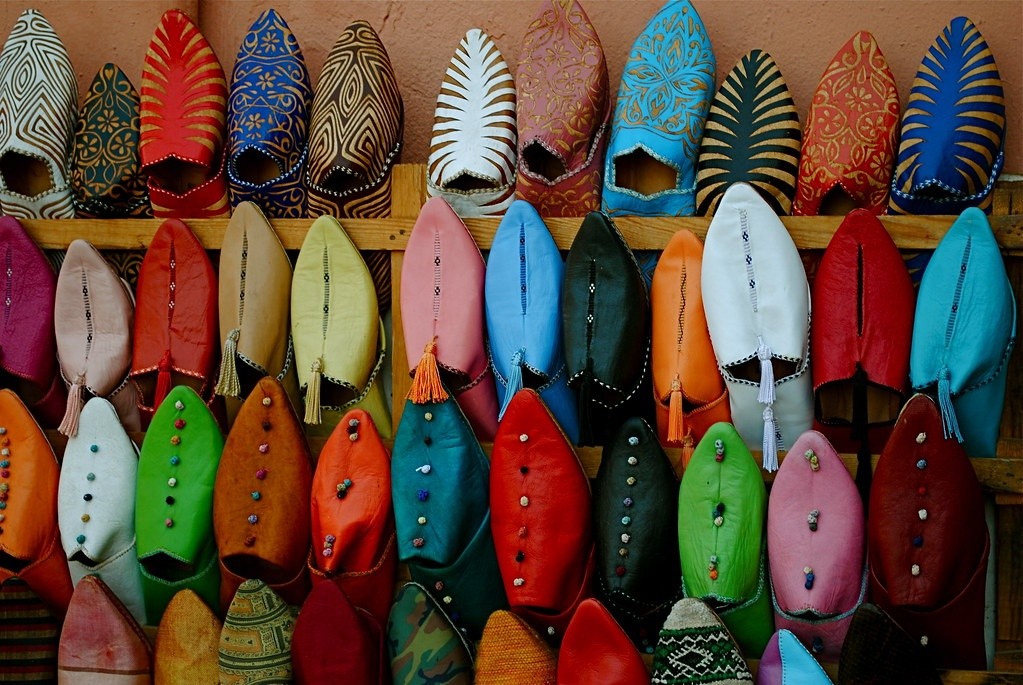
[{"xmin": 0, "ymin": 1, "xmax": 1017, "ymax": 685}]
